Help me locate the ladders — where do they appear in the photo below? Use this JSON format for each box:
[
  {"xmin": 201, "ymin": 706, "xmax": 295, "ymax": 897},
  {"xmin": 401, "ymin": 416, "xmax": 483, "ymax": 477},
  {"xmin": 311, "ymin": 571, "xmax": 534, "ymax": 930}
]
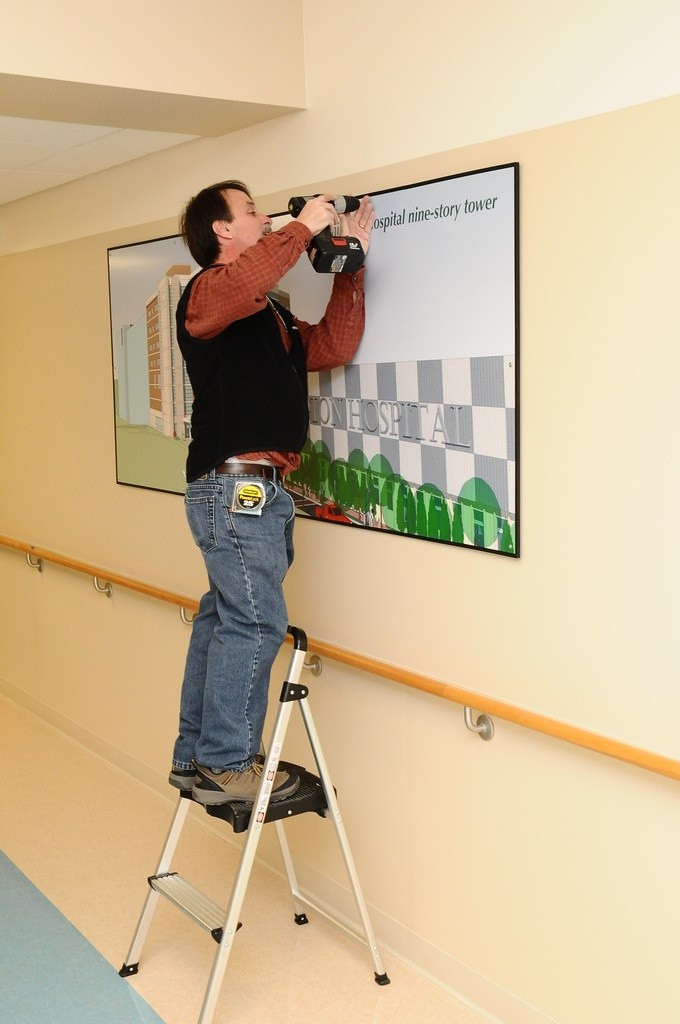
[{"xmin": 121, "ymin": 622, "xmax": 389, "ymax": 1023}]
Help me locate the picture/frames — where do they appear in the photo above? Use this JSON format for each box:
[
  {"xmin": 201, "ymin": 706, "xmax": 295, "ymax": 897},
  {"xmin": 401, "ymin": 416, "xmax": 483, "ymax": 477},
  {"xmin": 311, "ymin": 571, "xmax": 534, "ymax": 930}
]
[{"xmin": 106, "ymin": 163, "xmax": 522, "ymax": 557}]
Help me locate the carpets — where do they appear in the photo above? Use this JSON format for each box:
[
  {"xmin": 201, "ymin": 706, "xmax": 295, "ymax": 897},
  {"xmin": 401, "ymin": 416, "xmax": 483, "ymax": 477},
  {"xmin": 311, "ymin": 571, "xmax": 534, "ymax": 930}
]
[{"xmin": 0, "ymin": 849, "xmax": 167, "ymax": 1024}]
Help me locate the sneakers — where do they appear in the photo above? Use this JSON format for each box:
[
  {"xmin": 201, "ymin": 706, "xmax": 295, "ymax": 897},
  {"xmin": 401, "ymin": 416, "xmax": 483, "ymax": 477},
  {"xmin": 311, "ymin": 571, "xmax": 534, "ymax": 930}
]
[
  {"xmin": 193, "ymin": 758, "xmax": 301, "ymax": 803},
  {"xmin": 168, "ymin": 772, "xmax": 193, "ymax": 791}
]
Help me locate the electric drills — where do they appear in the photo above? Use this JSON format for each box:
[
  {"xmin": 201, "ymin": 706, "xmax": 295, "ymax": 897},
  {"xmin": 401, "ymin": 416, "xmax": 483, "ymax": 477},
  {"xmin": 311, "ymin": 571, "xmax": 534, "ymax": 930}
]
[{"xmin": 286, "ymin": 193, "xmax": 367, "ymax": 273}]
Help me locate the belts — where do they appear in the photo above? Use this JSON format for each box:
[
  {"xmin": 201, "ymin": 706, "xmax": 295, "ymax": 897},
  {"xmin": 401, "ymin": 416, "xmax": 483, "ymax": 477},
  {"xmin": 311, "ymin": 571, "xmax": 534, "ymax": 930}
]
[{"xmin": 213, "ymin": 464, "xmax": 282, "ymax": 481}]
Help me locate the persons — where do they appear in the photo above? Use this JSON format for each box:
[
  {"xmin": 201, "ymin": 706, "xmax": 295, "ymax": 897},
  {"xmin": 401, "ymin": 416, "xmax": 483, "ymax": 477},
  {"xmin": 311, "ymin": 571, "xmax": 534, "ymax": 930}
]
[{"xmin": 168, "ymin": 179, "xmax": 377, "ymax": 807}]
